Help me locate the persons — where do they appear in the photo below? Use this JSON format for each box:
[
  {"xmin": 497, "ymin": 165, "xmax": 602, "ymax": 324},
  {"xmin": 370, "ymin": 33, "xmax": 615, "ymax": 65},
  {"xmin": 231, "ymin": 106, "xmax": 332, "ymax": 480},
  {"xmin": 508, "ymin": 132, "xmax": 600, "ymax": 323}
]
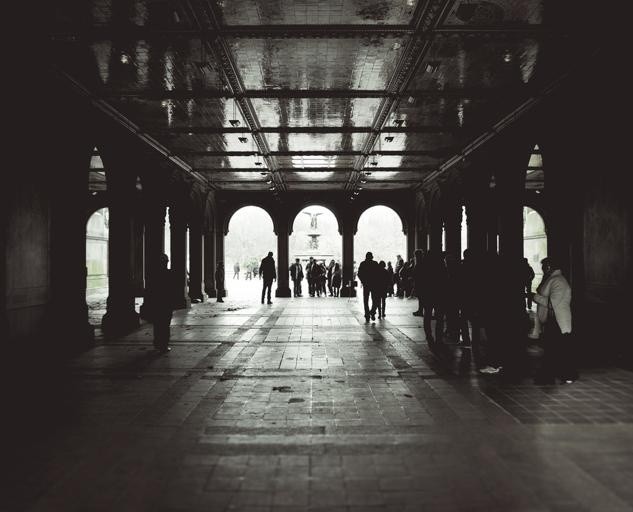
[
  {"xmin": 253, "ymin": 265, "xmax": 258, "ymax": 278},
  {"xmin": 258, "ymin": 252, "xmax": 276, "ymax": 304},
  {"xmin": 358, "ymin": 248, "xmax": 579, "ymax": 384},
  {"xmin": 289, "ymin": 257, "xmax": 341, "ymax": 297},
  {"xmin": 150, "ymin": 254, "xmax": 175, "ymax": 356},
  {"xmin": 215, "ymin": 262, "xmax": 224, "ymax": 303},
  {"xmin": 245, "ymin": 262, "xmax": 252, "ymax": 280},
  {"xmin": 233, "ymin": 262, "xmax": 240, "ymax": 279}
]
